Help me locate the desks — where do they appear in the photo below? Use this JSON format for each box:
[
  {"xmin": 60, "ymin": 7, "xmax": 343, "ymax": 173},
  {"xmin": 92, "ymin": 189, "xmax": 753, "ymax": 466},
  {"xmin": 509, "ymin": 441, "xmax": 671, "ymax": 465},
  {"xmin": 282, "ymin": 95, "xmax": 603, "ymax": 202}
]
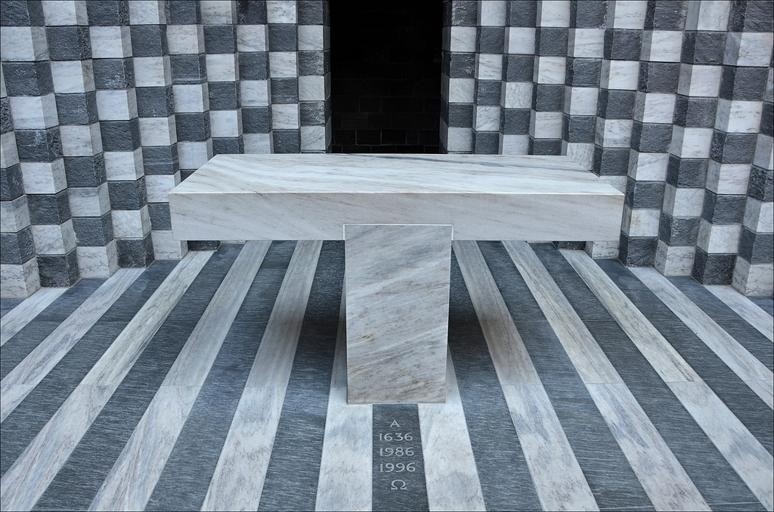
[{"xmin": 164, "ymin": 151, "xmax": 625, "ymax": 406}]
